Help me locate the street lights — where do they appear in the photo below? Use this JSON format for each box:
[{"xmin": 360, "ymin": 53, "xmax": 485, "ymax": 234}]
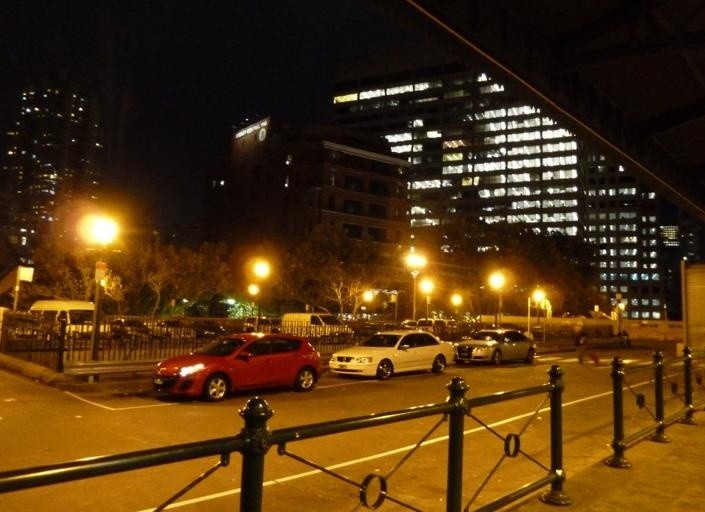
[
  {"xmin": 81, "ymin": 209, "xmax": 119, "ymax": 384},
  {"xmin": 489, "ymin": 271, "xmax": 503, "ymax": 325},
  {"xmin": 245, "ymin": 258, "xmax": 271, "ymax": 334},
  {"xmin": 405, "ymin": 255, "xmax": 430, "ymax": 320},
  {"xmin": 451, "ymin": 295, "xmax": 463, "ymax": 315},
  {"xmin": 524, "ymin": 288, "xmax": 544, "ymax": 331},
  {"xmin": 617, "ymin": 302, "xmax": 626, "ymax": 311},
  {"xmin": 248, "ymin": 283, "xmax": 260, "ymax": 314},
  {"xmin": 423, "ymin": 282, "xmax": 433, "ymax": 318}
]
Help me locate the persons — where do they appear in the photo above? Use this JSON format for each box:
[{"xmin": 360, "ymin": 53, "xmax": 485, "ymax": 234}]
[{"xmin": 573, "ymin": 321, "xmax": 600, "ymax": 365}]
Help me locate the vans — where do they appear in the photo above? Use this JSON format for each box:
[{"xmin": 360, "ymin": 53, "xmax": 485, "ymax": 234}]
[
  {"xmin": 29, "ymin": 299, "xmax": 111, "ymax": 343},
  {"xmin": 243, "ymin": 317, "xmax": 281, "ymax": 334},
  {"xmin": 281, "ymin": 312, "xmax": 354, "ymax": 342}
]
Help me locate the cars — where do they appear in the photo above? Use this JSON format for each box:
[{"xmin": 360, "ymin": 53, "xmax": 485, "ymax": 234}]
[
  {"xmin": 365, "ymin": 318, "xmax": 459, "ymax": 334},
  {"xmin": 109, "ymin": 317, "xmax": 158, "ymax": 348},
  {"xmin": 190, "ymin": 319, "xmax": 225, "ymax": 340},
  {"xmin": 329, "ymin": 328, "xmax": 457, "ymax": 378},
  {"xmin": 155, "ymin": 320, "xmax": 196, "ymax": 342},
  {"xmin": 153, "ymin": 334, "xmax": 323, "ymax": 403},
  {"xmin": 2, "ymin": 310, "xmax": 70, "ymax": 353},
  {"xmin": 454, "ymin": 330, "xmax": 537, "ymax": 364}
]
